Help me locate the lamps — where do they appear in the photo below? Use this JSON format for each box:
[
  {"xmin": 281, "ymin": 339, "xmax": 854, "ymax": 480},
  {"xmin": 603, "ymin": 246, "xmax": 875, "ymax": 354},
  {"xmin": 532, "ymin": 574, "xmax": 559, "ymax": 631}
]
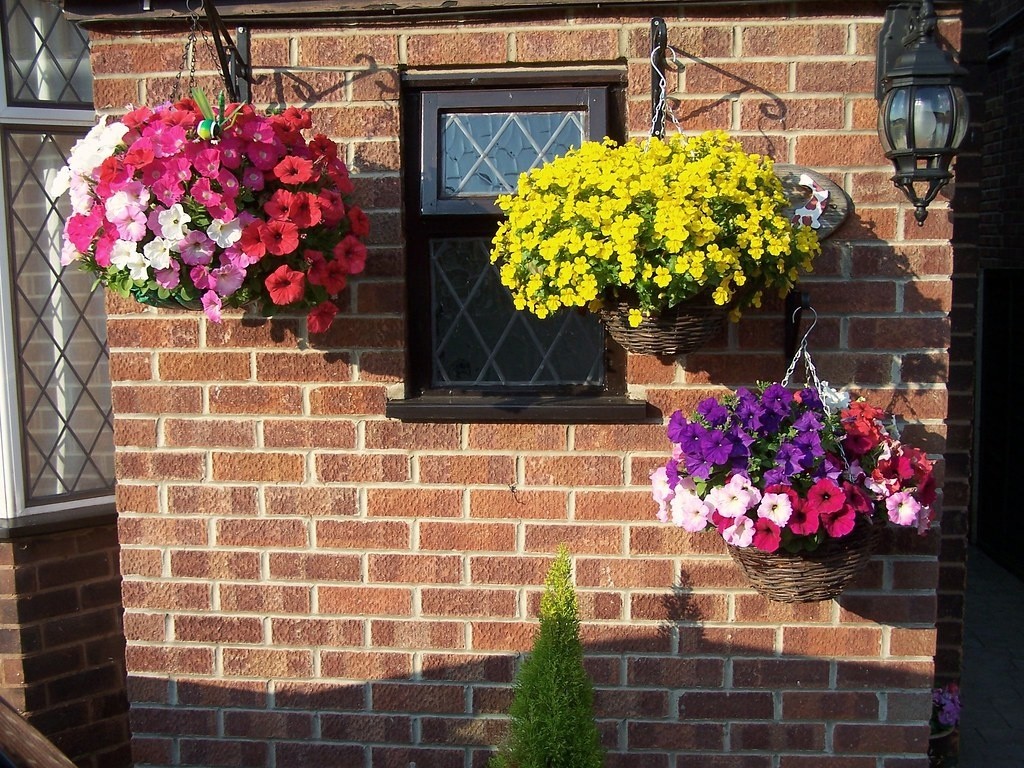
[{"xmin": 875, "ymin": 0, "xmax": 971, "ymax": 225}]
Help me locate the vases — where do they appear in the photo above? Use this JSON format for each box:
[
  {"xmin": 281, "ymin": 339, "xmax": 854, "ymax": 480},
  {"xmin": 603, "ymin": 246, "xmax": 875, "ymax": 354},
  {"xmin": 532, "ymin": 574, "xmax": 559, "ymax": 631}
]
[{"xmin": 929, "ymin": 723, "xmax": 956, "ymax": 748}]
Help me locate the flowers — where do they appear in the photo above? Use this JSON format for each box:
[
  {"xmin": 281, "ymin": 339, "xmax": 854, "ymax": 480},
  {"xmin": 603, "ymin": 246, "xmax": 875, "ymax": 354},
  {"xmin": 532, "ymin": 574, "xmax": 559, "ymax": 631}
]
[
  {"xmin": 930, "ymin": 687, "xmax": 961, "ymax": 735},
  {"xmin": 649, "ymin": 379, "xmax": 938, "ymax": 554},
  {"xmin": 56, "ymin": 90, "xmax": 368, "ymax": 332},
  {"xmin": 489, "ymin": 130, "xmax": 822, "ymax": 327}
]
